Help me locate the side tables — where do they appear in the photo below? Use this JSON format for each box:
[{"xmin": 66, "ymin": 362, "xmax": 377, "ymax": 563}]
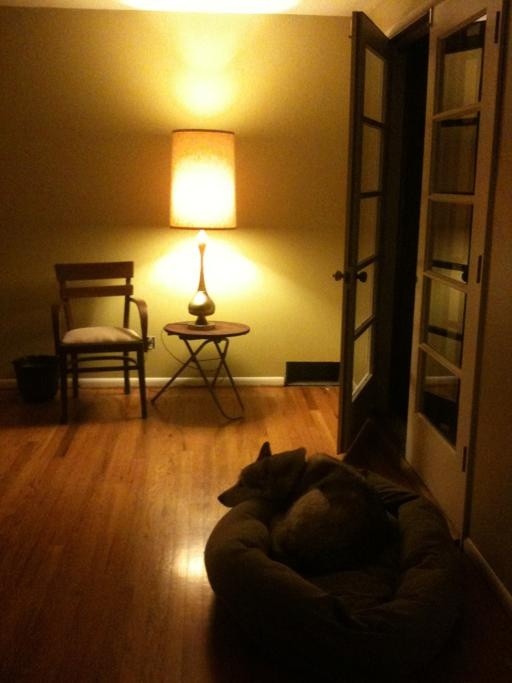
[{"xmin": 154, "ymin": 321, "xmax": 252, "ymax": 425}]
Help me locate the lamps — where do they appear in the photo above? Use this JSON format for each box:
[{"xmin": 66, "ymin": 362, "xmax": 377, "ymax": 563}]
[{"xmin": 167, "ymin": 129, "xmax": 239, "ymax": 328}]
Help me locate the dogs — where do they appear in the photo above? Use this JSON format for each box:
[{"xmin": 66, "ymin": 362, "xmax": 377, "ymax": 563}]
[{"xmin": 217, "ymin": 441, "xmax": 391, "ymax": 580}]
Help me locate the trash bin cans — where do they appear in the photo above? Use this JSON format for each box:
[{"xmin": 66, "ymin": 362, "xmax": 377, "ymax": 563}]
[{"xmin": 12, "ymin": 354, "xmax": 62, "ymax": 404}]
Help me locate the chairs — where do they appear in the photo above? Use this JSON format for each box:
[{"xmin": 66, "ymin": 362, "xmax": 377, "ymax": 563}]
[{"xmin": 49, "ymin": 260, "xmax": 148, "ymax": 425}]
